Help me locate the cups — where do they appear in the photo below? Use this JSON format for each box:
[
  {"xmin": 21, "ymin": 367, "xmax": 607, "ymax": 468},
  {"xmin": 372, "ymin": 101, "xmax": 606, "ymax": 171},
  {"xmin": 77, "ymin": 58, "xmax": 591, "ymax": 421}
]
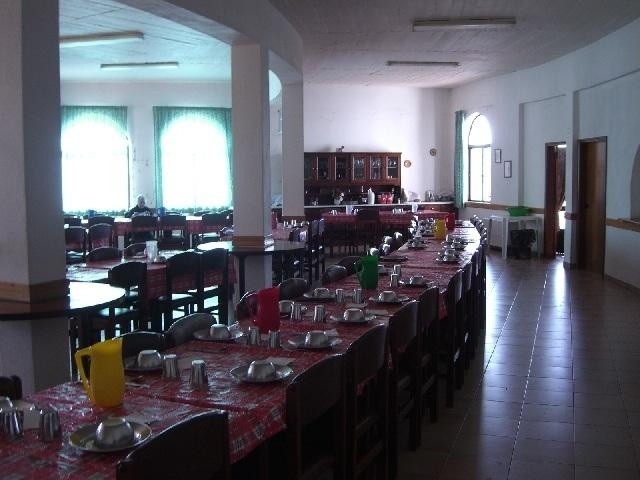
[
  {"xmin": 146, "ymin": 240, "xmax": 158, "ymax": 263},
  {"xmin": 183, "ymin": 263, "xmax": 427, "ymax": 350},
  {"xmin": 247, "ymin": 360, "xmax": 278, "ymax": 380},
  {"xmin": 0, "ymin": 404, "xmax": 25, "ymax": 441},
  {"xmin": 34, "ymin": 407, "xmax": 63, "ymax": 444},
  {"xmin": 189, "ymin": 360, "xmax": 209, "ymax": 389},
  {"xmin": 291, "ymin": 219, "xmax": 297, "ymax": 227},
  {"xmin": 94, "ymin": 418, "xmax": 135, "ymax": 447},
  {"xmin": 161, "ymin": 352, "xmax": 180, "ymax": 382},
  {"xmin": 284, "ymin": 220, "xmax": 288, "ymax": 227},
  {"xmin": 139, "ymin": 350, "xmax": 162, "ymax": 367}
]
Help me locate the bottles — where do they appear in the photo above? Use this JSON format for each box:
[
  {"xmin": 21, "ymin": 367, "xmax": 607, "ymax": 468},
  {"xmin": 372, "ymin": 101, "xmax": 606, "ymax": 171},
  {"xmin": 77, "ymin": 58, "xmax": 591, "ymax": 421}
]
[
  {"xmin": 347, "ymin": 188, "xmax": 352, "ymax": 201},
  {"xmin": 391, "ymin": 186, "xmax": 406, "ymax": 204},
  {"xmin": 336, "ymin": 159, "xmax": 346, "ymax": 179},
  {"xmin": 355, "ymin": 176, "xmax": 365, "ymax": 181},
  {"xmin": 75, "ymin": 338, "xmax": 127, "ymax": 407},
  {"xmin": 358, "ymin": 186, "xmax": 367, "ymax": 204}
]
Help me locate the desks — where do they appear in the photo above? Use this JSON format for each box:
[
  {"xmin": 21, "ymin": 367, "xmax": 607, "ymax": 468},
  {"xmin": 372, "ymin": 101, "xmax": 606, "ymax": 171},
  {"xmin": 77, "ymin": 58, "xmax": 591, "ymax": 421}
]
[{"xmin": 486, "ymin": 214, "xmax": 542, "ymax": 261}]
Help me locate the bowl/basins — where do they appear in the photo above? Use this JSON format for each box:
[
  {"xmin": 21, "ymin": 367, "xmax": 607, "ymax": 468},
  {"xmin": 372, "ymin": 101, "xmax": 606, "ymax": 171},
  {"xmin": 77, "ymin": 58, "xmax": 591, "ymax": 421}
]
[{"xmin": 153, "ymin": 256, "xmax": 166, "ymax": 263}]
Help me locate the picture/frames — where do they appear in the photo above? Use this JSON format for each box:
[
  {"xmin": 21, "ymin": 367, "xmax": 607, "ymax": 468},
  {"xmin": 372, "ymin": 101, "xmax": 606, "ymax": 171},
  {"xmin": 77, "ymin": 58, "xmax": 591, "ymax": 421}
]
[
  {"xmin": 495, "ymin": 149, "xmax": 501, "ymax": 163},
  {"xmin": 504, "ymin": 160, "xmax": 513, "ymax": 178}
]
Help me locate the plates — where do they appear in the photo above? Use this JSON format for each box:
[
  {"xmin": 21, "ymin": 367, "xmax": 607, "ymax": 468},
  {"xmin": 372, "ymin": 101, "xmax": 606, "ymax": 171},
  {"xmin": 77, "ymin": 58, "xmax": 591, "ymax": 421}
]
[
  {"xmin": 228, "ymin": 362, "xmax": 293, "ymax": 385},
  {"xmin": 405, "ymin": 219, "xmax": 467, "ymax": 263},
  {"xmin": 64, "ymin": 419, "xmax": 152, "ymax": 451},
  {"xmin": 380, "ymin": 256, "xmax": 408, "ymax": 261},
  {"xmin": 126, "ymin": 356, "xmax": 163, "ymax": 372}
]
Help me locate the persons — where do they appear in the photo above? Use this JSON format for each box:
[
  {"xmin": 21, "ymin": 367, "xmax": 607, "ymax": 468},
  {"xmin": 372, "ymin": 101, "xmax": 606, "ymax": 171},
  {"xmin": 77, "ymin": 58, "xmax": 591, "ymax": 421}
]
[{"xmin": 125, "ymin": 195, "xmax": 153, "ymax": 240}]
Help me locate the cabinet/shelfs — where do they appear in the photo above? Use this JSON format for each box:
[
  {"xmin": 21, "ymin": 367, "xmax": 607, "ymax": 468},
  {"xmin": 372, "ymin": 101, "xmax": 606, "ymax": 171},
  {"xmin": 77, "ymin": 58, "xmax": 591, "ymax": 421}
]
[{"xmin": 304, "ymin": 151, "xmax": 402, "ymax": 204}]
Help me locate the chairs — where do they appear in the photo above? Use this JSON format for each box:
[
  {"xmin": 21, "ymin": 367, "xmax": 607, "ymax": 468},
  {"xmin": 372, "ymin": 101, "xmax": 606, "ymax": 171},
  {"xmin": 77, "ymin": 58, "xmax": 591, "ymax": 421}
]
[
  {"xmin": 436, "ymin": 269, "xmax": 464, "ymax": 375},
  {"xmin": 419, "ymin": 285, "xmax": 440, "ymax": 449},
  {"xmin": 463, "ymin": 260, "xmax": 473, "ymax": 354},
  {"xmin": 265, "ymin": 353, "xmax": 347, "ymax": 480},
  {"xmin": 116, "ymin": 409, "xmax": 231, "ymax": 480},
  {"xmin": 346, "ymin": 324, "xmax": 390, "ymax": 479},
  {"xmin": 469, "ymin": 213, "xmax": 488, "ymax": 281},
  {"xmin": 388, "ymin": 299, "xmax": 420, "ymax": 478}
]
